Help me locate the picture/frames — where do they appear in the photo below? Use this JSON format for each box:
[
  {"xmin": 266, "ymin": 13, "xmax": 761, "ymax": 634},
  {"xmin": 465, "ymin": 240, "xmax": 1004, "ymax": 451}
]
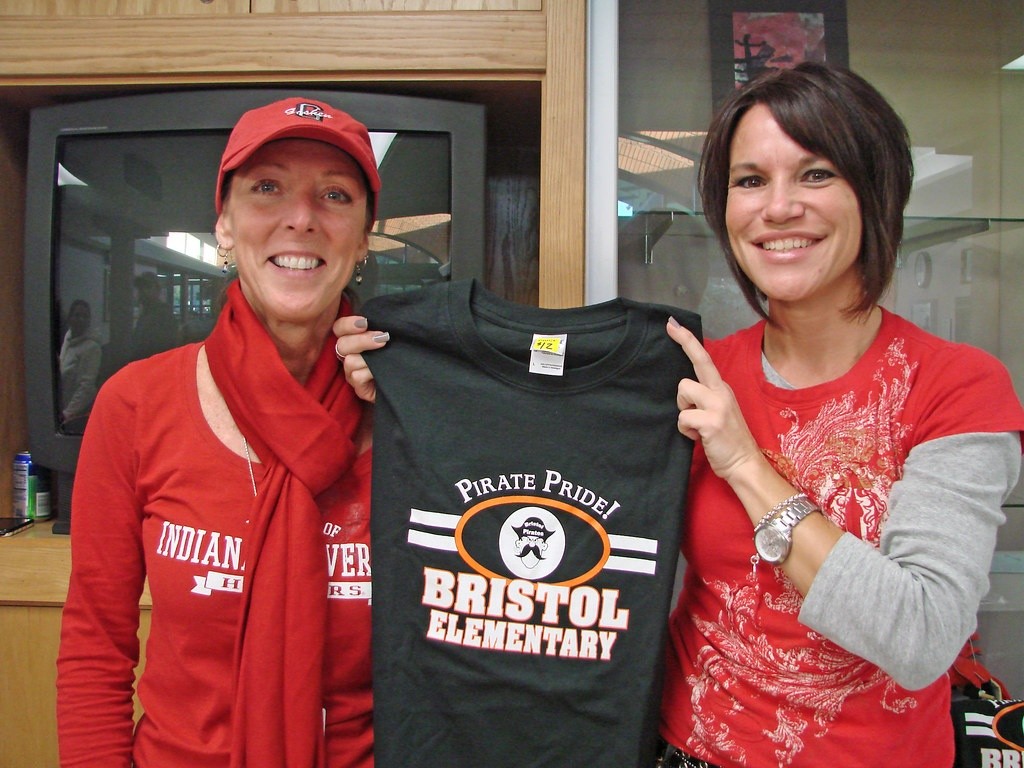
[{"xmin": 707, "ymin": 0, "xmax": 849, "ymax": 117}]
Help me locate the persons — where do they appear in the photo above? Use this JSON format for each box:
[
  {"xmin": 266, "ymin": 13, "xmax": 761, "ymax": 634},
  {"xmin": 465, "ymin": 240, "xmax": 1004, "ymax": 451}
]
[
  {"xmin": 55, "ymin": 97, "xmax": 375, "ymax": 768},
  {"xmin": 329, "ymin": 60, "xmax": 1024, "ymax": 768},
  {"xmin": 130, "ymin": 271, "xmax": 177, "ymax": 361},
  {"xmin": 57, "ymin": 299, "xmax": 103, "ymax": 421}
]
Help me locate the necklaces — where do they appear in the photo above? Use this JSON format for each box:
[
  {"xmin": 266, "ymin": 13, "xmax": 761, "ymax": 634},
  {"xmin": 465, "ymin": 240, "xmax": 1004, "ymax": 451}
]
[{"xmin": 243, "ymin": 436, "xmax": 258, "ymax": 496}]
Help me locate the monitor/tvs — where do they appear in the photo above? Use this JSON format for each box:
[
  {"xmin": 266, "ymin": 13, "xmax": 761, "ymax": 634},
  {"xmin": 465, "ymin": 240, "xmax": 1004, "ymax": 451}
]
[{"xmin": 25, "ymin": 92, "xmax": 488, "ymax": 535}]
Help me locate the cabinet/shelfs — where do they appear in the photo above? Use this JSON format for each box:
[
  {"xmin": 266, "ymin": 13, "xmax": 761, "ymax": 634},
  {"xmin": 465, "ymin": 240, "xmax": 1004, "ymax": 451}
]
[
  {"xmin": 0, "ymin": 0, "xmax": 587, "ymax": 768},
  {"xmin": 620, "ymin": 209, "xmax": 1024, "ymax": 509}
]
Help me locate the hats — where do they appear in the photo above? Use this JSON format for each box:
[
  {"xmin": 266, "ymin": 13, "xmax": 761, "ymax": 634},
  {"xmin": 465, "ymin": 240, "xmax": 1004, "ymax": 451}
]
[{"xmin": 216, "ymin": 97, "xmax": 380, "ymax": 232}]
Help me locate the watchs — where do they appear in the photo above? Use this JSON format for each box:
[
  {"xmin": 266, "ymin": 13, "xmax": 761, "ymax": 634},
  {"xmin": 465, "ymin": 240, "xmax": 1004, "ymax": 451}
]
[{"xmin": 754, "ymin": 498, "xmax": 822, "ymax": 565}]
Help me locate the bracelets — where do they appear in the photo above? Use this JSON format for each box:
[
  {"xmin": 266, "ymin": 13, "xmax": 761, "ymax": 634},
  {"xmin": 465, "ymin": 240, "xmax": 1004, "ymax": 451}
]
[{"xmin": 754, "ymin": 492, "xmax": 809, "ymax": 531}]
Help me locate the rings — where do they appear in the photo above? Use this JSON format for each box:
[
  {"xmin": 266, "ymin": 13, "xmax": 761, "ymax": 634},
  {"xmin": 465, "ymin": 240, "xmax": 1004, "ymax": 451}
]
[{"xmin": 335, "ymin": 344, "xmax": 345, "ymax": 361}]
